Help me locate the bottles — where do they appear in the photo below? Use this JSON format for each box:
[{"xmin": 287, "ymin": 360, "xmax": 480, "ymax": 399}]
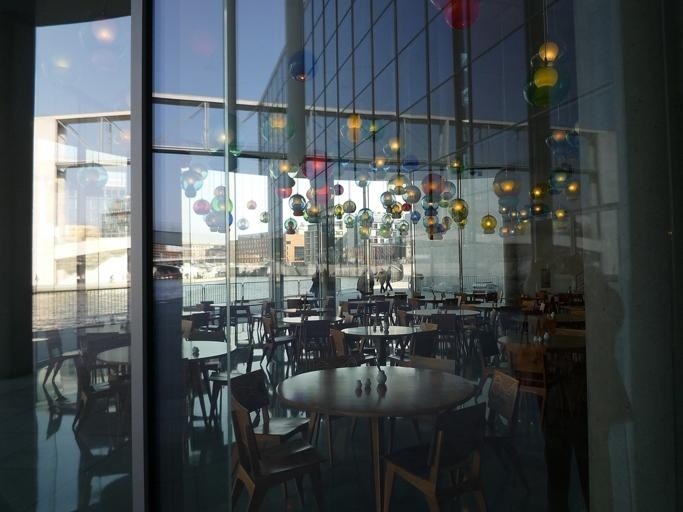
[
  {"xmin": 355, "ymin": 370, "xmax": 387, "ymax": 389},
  {"xmin": 192, "ymin": 347, "xmax": 199, "ymax": 354},
  {"xmin": 373, "ymin": 322, "xmax": 389, "ymax": 331},
  {"xmin": 547, "ymin": 312, "xmax": 556, "ymax": 319},
  {"xmin": 533, "ymin": 332, "xmax": 550, "ymax": 343}
]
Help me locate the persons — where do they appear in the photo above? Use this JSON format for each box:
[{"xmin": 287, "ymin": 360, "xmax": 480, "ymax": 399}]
[
  {"xmin": 308, "ymin": 268, "xmax": 319, "ymax": 297},
  {"xmin": 376, "ymin": 268, "xmax": 387, "ymax": 293},
  {"xmin": 361, "ymin": 267, "xmax": 374, "ymax": 296},
  {"xmin": 384, "ymin": 266, "xmax": 393, "ymax": 291},
  {"xmin": 522, "ymin": 217, "xmax": 634, "ymax": 511}
]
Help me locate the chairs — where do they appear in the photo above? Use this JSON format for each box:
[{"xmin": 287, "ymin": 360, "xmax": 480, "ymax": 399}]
[
  {"xmin": 42, "ymin": 329, "xmax": 87, "ymax": 407},
  {"xmin": 45, "ymin": 406, "xmax": 64, "ymax": 440}
]
